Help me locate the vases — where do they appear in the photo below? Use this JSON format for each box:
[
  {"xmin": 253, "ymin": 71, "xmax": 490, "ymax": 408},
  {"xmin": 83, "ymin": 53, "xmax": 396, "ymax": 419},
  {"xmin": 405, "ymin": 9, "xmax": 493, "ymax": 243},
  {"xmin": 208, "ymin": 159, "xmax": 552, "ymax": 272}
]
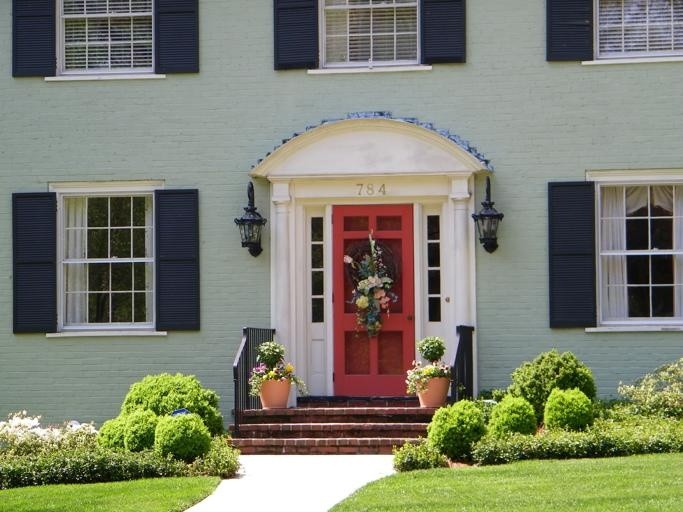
[
  {"xmin": 415, "ymin": 377, "xmax": 448, "ymax": 408},
  {"xmin": 257, "ymin": 380, "xmax": 290, "ymax": 410}
]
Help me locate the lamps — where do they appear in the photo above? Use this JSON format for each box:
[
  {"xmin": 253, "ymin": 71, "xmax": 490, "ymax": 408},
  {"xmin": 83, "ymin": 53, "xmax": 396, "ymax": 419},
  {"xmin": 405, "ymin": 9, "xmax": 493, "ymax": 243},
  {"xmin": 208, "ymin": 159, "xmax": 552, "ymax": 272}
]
[
  {"xmin": 232, "ymin": 182, "xmax": 267, "ymax": 258},
  {"xmin": 471, "ymin": 176, "xmax": 503, "ymax": 254}
]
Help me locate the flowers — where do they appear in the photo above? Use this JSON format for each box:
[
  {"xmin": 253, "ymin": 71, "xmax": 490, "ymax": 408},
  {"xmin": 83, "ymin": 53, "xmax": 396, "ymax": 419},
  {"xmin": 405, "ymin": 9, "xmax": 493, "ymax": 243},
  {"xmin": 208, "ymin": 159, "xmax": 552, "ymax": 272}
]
[
  {"xmin": 341, "ymin": 229, "xmax": 398, "ymax": 337},
  {"xmin": 411, "ymin": 334, "xmax": 445, "ymax": 362},
  {"xmin": 254, "ymin": 342, "xmax": 283, "ymax": 365},
  {"xmin": 404, "ymin": 358, "xmax": 454, "ymax": 395},
  {"xmin": 247, "ymin": 363, "xmax": 308, "ymax": 402}
]
